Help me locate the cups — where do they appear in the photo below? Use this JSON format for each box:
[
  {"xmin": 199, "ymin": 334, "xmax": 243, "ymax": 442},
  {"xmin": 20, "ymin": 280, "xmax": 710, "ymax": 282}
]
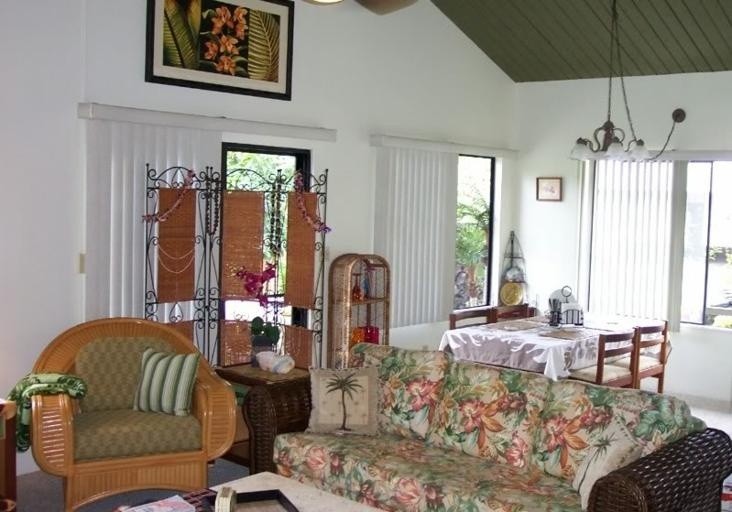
[{"xmin": 256, "ymin": 351, "xmax": 279, "ymax": 372}]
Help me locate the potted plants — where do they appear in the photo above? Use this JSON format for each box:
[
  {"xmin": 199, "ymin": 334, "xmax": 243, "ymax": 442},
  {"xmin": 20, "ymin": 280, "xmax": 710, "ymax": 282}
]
[{"xmin": 250, "ymin": 313, "xmax": 283, "ymax": 369}]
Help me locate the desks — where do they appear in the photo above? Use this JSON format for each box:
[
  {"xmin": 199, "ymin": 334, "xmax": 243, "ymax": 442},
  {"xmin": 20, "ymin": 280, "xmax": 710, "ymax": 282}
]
[
  {"xmin": 0, "ymin": 398, "xmax": 18, "ymax": 512},
  {"xmin": 438, "ymin": 314, "xmax": 662, "ymax": 381}
]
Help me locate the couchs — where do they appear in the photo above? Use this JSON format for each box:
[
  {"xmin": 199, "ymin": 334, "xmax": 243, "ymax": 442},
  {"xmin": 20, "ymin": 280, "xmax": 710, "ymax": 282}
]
[{"xmin": 272, "ymin": 336, "xmax": 731, "ymax": 512}]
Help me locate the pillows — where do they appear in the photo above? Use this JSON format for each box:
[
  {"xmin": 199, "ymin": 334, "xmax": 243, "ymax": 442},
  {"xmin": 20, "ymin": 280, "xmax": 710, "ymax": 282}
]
[
  {"xmin": 132, "ymin": 344, "xmax": 201, "ymax": 418},
  {"xmin": 573, "ymin": 414, "xmax": 646, "ymax": 511},
  {"xmin": 308, "ymin": 368, "xmax": 379, "ymax": 435}
]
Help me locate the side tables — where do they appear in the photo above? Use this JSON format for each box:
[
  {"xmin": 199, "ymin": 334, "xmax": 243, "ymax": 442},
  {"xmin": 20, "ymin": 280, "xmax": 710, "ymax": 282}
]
[{"xmin": 217, "ymin": 352, "xmax": 312, "ymax": 472}]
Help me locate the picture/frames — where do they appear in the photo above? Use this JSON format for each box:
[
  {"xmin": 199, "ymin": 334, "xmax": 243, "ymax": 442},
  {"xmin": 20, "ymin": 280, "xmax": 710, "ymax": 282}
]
[
  {"xmin": 536, "ymin": 177, "xmax": 562, "ymax": 202},
  {"xmin": 146, "ymin": 0, "xmax": 294, "ymax": 101}
]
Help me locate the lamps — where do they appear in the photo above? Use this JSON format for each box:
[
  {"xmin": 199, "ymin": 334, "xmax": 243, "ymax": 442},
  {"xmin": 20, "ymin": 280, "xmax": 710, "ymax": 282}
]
[{"xmin": 569, "ymin": 0, "xmax": 685, "ymax": 166}]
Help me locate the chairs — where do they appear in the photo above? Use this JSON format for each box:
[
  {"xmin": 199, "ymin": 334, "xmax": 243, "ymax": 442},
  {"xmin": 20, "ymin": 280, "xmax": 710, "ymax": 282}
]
[
  {"xmin": 27, "ymin": 320, "xmax": 241, "ymax": 512},
  {"xmin": 449, "ymin": 307, "xmax": 496, "ymax": 329},
  {"xmin": 569, "ymin": 326, "xmax": 640, "ymax": 388},
  {"xmin": 495, "ymin": 303, "xmax": 530, "ymax": 323},
  {"xmin": 638, "ymin": 321, "xmax": 670, "ymax": 393}
]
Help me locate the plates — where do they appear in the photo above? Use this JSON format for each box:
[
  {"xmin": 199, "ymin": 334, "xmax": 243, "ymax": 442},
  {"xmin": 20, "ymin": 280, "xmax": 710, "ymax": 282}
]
[{"xmin": 500, "ymin": 283, "xmax": 523, "ymax": 306}]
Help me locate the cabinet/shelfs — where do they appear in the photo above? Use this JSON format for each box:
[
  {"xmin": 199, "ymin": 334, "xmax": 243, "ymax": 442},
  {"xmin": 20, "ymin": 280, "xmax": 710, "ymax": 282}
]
[{"xmin": 328, "ymin": 251, "xmax": 392, "ymax": 368}]
